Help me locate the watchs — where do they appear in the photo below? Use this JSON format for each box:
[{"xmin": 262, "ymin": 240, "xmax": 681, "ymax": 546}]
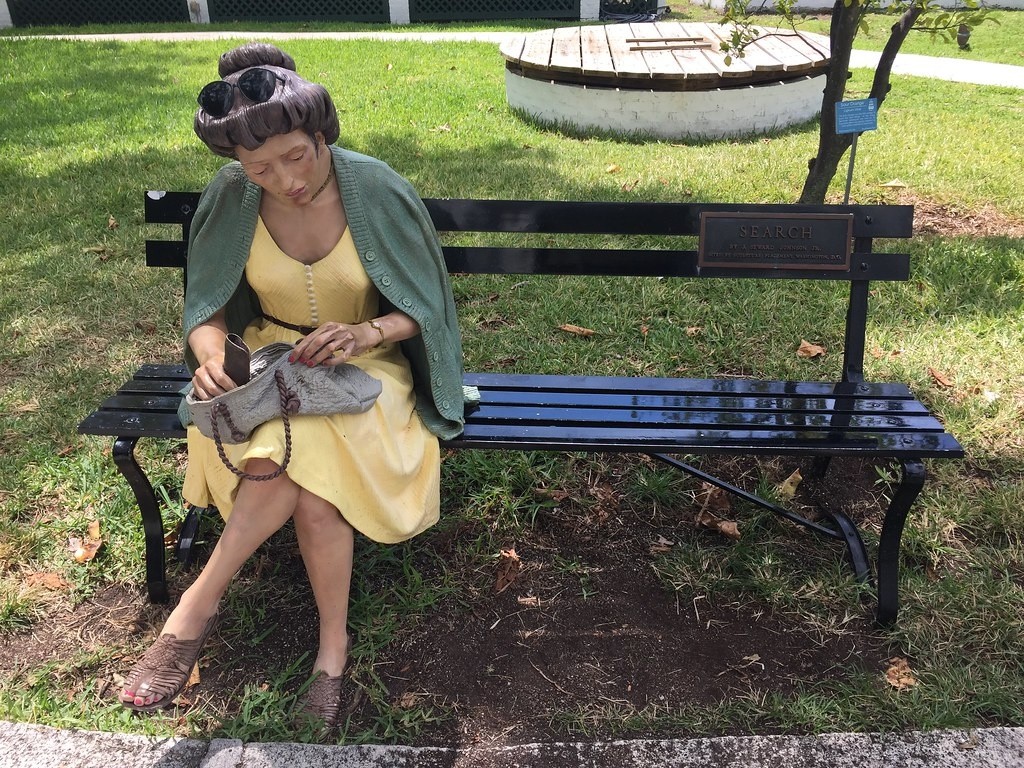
[{"xmin": 367, "ymin": 319, "xmax": 384, "ymax": 349}]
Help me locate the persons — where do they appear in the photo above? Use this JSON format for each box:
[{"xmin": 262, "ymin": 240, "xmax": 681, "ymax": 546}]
[{"xmin": 122, "ymin": 45, "xmax": 466, "ymax": 741}]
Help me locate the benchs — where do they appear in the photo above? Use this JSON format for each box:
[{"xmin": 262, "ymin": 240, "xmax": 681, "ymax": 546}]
[{"xmin": 74, "ymin": 187, "xmax": 966, "ymax": 629}]
